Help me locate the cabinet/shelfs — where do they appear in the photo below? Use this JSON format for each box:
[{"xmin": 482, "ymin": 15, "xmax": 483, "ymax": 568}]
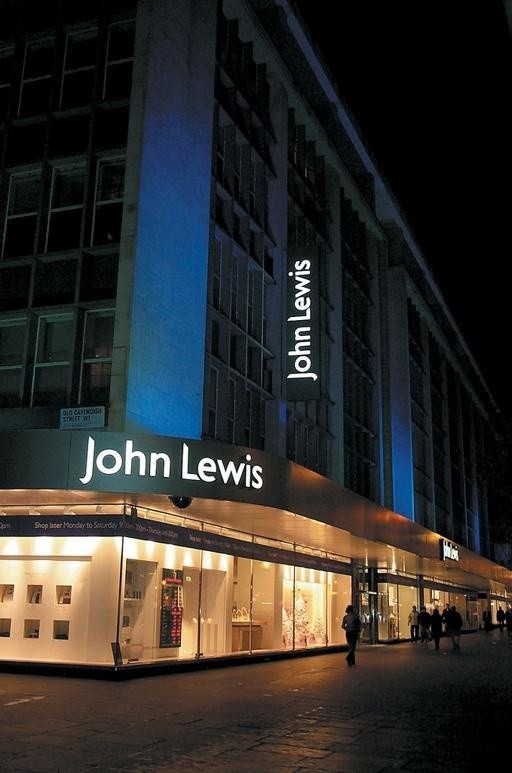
[
  {"xmin": 117, "ymin": 556, "xmax": 160, "ymax": 664},
  {"xmin": 231, "ymin": 618, "xmax": 267, "ymax": 652}
]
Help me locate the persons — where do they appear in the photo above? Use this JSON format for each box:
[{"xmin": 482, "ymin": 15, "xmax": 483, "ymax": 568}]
[
  {"xmin": 504, "ymin": 600, "xmax": 512, "ymax": 637},
  {"xmin": 406, "ymin": 605, "xmax": 419, "ymax": 642},
  {"xmin": 428, "ymin": 608, "xmax": 442, "ymax": 649},
  {"xmin": 341, "ymin": 604, "xmax": 361, "ymax": 666},
  {"xmin": 443, "ymin": 605, "xmax": 463, "ymax": 650},
  {"xmin": 417, "ymin": 606, "xmax": 431, "ymax": 644},
  {"xmin": 496, "ymin": 606, "xmax": 504, "ymax": 631},
  {"xmin": 442, "ymin": 602, "xmax": 450, "ymax": 637},
  {"xmin": 482, "ymin": 605, "xmax": 491, "ymax": 633}
]
